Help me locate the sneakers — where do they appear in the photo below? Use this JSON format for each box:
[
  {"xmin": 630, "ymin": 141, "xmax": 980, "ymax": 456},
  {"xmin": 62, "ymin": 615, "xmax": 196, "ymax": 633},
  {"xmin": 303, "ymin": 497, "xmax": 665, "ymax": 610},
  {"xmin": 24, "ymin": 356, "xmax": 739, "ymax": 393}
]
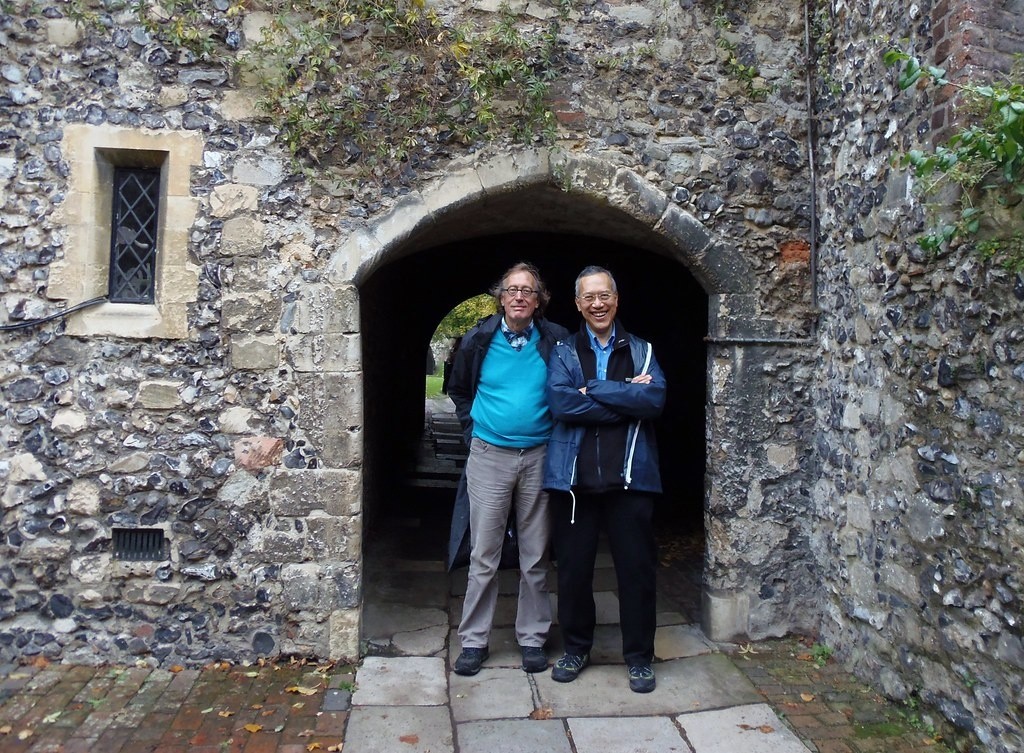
[
  {"xmin": 522, "ymin": 646, "xmax": 548, "ymax": 672},
  {"xmin": 552, "ymin": 652, "xmax": 591, "ymax": 682},
  {"xmin": 628, "ymin": 663, "xmax": 656, "ymax": 693},
  {"xmin": 454, "ymin": 646, "xmax": 489, "ymax": 674}
]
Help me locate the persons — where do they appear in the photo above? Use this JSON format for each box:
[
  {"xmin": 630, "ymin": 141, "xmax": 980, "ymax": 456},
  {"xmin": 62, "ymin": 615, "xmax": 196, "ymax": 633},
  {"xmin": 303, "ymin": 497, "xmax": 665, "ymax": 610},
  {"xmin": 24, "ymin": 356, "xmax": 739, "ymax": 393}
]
[
  {"xmin": 446, "ymin": 262, "xmax": 569, "ymax": 675},
  {"xmin": 542, "ymin": 266, "xmax": 667, "ymax": 693}
]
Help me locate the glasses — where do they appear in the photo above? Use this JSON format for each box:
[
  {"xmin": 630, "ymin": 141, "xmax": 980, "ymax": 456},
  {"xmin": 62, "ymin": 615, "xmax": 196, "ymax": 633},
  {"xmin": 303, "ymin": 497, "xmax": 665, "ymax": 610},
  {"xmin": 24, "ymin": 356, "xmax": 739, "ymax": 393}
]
[
  {"xmin": 578, "ymin": 293, "xmax": 613, "ymax": 301},
  {"xmin": 501, "ymin": 286, "xmax": 539, "ymax": 297}
]
